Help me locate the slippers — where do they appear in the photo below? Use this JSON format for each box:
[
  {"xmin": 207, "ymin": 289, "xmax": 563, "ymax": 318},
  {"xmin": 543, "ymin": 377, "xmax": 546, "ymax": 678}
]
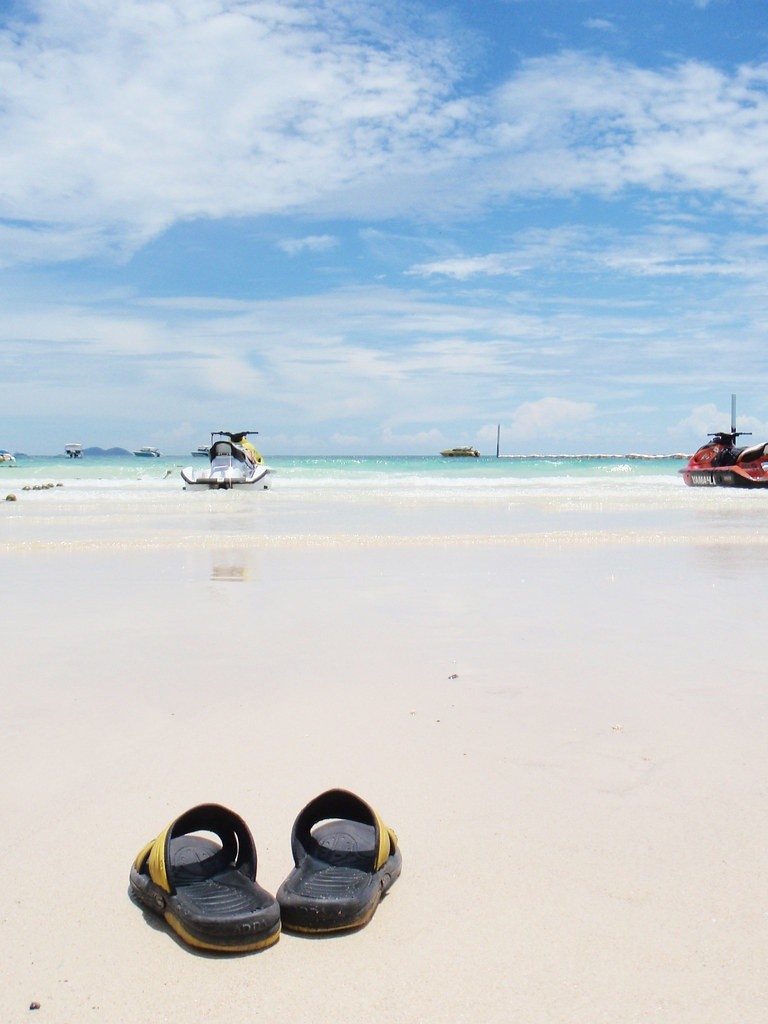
[{"xmin": 130, "ymin": 787, "xmax": 403, "ymax": 953}]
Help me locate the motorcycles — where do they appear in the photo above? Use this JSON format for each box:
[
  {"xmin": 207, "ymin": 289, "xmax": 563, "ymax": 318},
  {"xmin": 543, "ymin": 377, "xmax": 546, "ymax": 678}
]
[
  {"xmin": 179, "ymin": 431, "xmax": 277, "ymax": 491},
  {"xmin": 677, "ymin": 432, "xmax": 768, "ymax": 488}
]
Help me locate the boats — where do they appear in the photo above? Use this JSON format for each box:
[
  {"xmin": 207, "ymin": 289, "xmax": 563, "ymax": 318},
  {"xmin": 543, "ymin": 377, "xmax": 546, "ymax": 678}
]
[
  {"xmin": 439, "ymin": 446, "xmax": 480, "ymax": 458},
  {"xmin": 134, "ymin": 446, "xmax": 161, "ymax": 457},
  {"xmin": 191, "ymin": 445, "xmax": 211, "ymax": 458},
  {"xmin": 64, "ymin": 444, "xmax": 83, "ymax": 459}
]
